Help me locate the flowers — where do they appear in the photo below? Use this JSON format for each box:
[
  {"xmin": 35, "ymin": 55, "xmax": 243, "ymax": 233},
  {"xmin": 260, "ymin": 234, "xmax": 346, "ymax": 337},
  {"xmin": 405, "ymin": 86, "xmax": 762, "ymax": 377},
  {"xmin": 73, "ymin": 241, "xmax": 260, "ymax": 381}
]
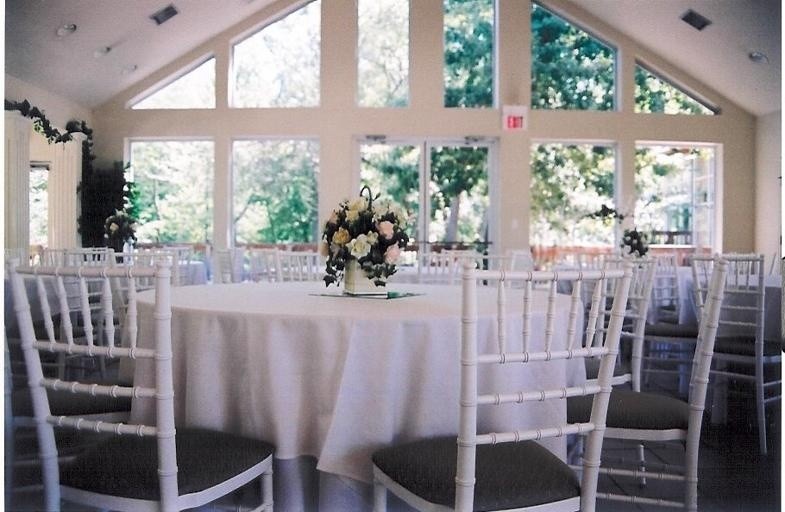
[
  {"xmin": 318, "ymin": 196, "xmax": 410, "ymax": 286},
  {"xmin": 620, "ymin": 226, "xmax": 650, "ymax": 256}
]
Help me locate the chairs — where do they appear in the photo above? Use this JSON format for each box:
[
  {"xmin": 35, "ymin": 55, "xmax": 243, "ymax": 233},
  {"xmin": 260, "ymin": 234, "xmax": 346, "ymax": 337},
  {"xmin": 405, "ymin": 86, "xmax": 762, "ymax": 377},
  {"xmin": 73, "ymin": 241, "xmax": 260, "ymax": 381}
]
[{"xmin": 4, "ymin": 243, "xmax": 780, "ymax": 512}]
